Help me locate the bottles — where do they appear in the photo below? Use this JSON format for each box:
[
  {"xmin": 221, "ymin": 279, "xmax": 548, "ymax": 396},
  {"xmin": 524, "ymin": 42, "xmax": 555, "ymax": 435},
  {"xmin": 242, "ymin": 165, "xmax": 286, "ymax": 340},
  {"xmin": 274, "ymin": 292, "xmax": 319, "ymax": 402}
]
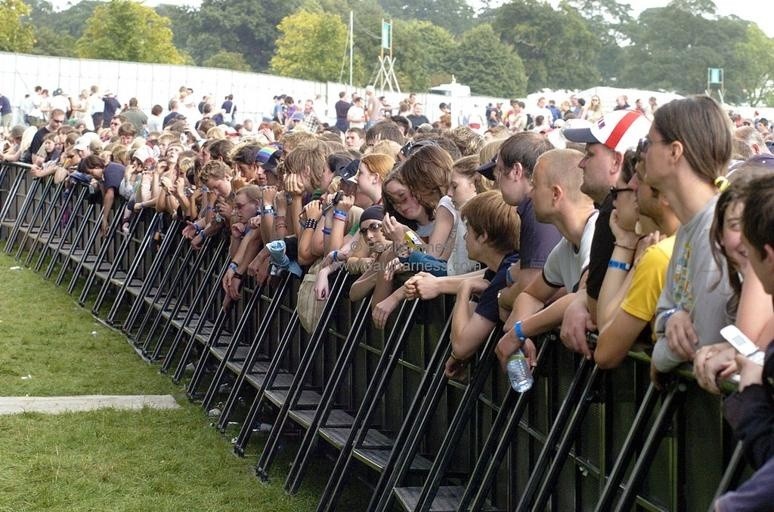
[{"xmin": 506, "ymin": 349, "xmax": 534, "ymax": 392}]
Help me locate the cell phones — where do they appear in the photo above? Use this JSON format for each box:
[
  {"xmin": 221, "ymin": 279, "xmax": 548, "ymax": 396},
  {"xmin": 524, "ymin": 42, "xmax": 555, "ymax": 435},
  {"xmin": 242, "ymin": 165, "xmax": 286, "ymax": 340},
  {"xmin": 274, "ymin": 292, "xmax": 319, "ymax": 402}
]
[
  {"xmin": 322, "ymin": 192, "xmax": 340, "ymax": 211},
  {"xmin": 299, "ymin": 202, "xmax": 322, "ymax": 223},
  {"xmin": 719, "ymin": 324, "xmax": 765, "ymax": 366}
]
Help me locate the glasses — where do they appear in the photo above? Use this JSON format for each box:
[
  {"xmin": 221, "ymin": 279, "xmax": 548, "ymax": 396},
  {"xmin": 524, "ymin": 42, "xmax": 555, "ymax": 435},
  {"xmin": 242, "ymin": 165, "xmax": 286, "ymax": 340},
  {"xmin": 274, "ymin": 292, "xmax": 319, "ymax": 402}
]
[
  {"xmin": 638, "ymin": 137, "xmax": 671, "ymax": 152},
  {"xmin": 610, "ymin": 186, "xmax": 633, "ymax": 200}
]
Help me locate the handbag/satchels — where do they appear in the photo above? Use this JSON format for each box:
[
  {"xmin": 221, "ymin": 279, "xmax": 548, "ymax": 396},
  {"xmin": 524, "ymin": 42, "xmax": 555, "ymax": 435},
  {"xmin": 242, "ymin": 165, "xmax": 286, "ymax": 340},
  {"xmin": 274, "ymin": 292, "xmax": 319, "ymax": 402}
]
[{"xmin": 296, "ymin": 260, "xmax": 329, "ymax": 335}]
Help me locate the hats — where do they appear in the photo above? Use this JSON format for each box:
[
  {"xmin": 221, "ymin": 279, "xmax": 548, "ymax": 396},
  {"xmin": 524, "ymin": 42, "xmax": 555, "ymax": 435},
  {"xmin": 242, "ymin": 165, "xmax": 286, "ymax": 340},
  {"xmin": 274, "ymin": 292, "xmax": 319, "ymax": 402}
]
[
  {"xmin": 291, "ymin": 112, "xmax": 304, "ymax": 121},
  {"xmin": 474, "ymin": 152, "xmax": 498, "ymax": 181},
  {"xmin": 546, "ymin": 118, "xmax": 593, "ymax": 150},
  {"xmin": 339, "ymin": 158, "xmax": 360, "ymax": 188},
  {"xmin": 563, "ymin": 109, "xmax": 652, "ymax": 158},
  {"xmin": 254, "ymin": 144, "xmax": 285, "ymax": 172},
  {"xmin": 359, "ymin": 204, "xmax": 384, "ymax": 227},
  {"xmin": 131, "ymin": 145, "xmax": 154, "ymax": 164},
  {"xmin": 71, "ymin": 136, "xmax": 90, "ymax": 151},
  {"xmin": 729, "ymin": 152, "xmax": 774, "ymax": 169}
]
